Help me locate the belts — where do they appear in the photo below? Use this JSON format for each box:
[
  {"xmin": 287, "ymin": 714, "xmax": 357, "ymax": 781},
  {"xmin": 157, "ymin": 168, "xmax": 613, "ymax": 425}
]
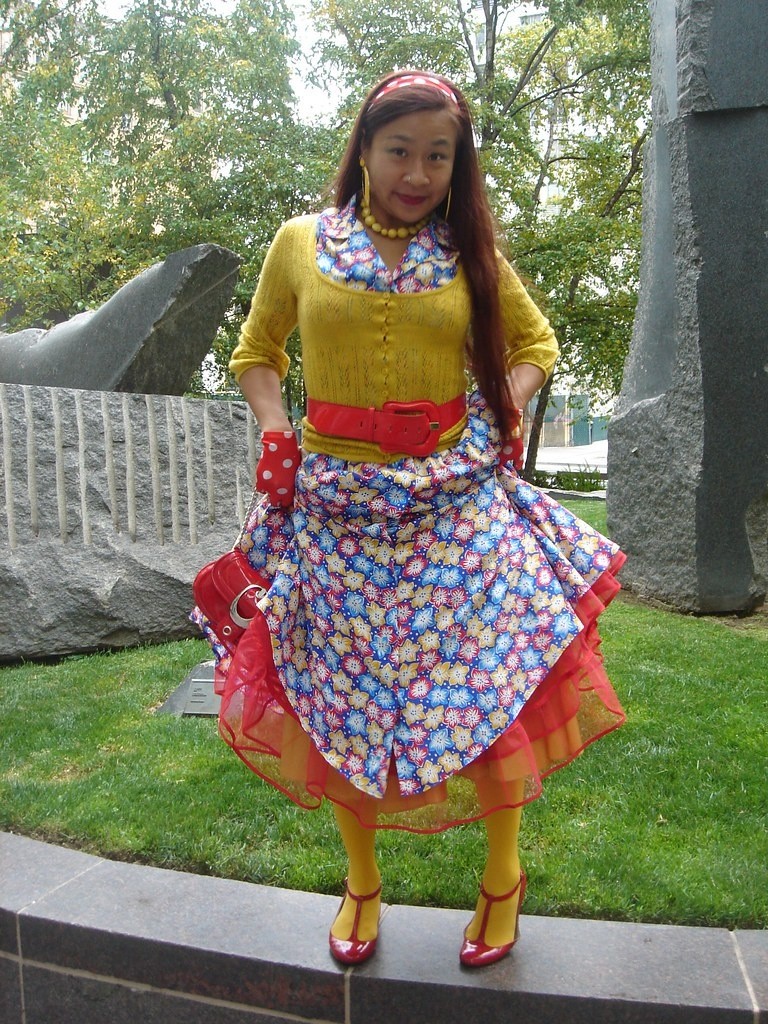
[{"xmin": 306, "ymin": 393, "xmax": 468, "ymax": 456}]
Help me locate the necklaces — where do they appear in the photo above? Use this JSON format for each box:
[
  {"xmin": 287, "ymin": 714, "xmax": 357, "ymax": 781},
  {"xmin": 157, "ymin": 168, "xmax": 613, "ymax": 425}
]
[{"xmin": 361, "ymin": 197, "xmax": 434, "ymax": 239}]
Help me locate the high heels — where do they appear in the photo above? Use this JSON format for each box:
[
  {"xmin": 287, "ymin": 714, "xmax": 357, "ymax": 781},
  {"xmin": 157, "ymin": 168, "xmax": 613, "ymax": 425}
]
[
  {"xmin": 329, "ymin": 876, "xmax": 382, "ymax": 964},
  {"xmin": 459, "ymin": 867, "xmax": 526, "ymax": 966}
]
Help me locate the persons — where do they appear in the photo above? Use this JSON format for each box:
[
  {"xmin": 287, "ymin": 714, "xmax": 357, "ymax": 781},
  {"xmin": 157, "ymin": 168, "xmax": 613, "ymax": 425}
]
[{"xmin": 190, "ymin": 71, "xmax": 627, "ymax": 964}]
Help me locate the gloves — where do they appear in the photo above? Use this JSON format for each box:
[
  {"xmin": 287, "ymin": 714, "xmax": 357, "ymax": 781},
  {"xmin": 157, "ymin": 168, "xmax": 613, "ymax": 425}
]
[
  {"xmin": 256, "ymin": 432, "xmax": 302, "ymax": 508},
  {"xmin": 498, "ymin": 405, "xmax": 524, "ymax": 473}
]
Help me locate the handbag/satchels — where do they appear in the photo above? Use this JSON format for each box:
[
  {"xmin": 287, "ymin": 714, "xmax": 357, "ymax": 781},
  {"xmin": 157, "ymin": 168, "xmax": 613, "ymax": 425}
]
[{"xmin": 192, "ymin": 488, "xmax": 274, "ymax": 670}]
